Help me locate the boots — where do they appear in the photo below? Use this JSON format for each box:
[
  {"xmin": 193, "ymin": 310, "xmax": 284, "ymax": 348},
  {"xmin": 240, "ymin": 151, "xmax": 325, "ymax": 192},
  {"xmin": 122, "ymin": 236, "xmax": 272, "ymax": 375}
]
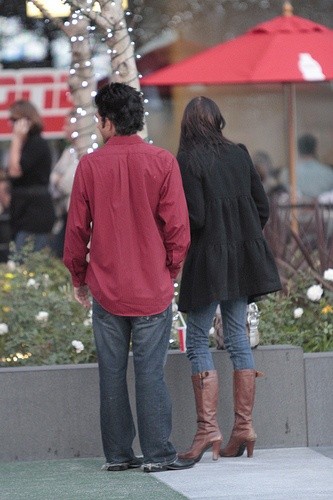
[
  {"xmin": 219, "ymin": 370, "xmax": 263, "ymax": 457},
  {"xmin": 178, "ymin": 370, "xmax": 224, "ymax": 461}
]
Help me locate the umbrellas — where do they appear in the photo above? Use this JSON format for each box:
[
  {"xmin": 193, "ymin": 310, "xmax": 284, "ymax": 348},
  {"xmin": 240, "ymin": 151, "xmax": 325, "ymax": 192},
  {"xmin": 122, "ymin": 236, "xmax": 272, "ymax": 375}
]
[{"xmin": 140, "ymin": 0, "xmax": 333, "ymax": 245}]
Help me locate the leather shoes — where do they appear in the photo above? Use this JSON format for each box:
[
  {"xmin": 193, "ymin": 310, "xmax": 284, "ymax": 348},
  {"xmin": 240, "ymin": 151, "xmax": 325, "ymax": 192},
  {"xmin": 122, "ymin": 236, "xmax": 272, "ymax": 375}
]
[
  {"xmin": 144, "ymin": 456, "xmax": 195, "ymax": 472},
  {"xmin": 107, "ymin": 456, "xmax": 142, "ymax": 471}
]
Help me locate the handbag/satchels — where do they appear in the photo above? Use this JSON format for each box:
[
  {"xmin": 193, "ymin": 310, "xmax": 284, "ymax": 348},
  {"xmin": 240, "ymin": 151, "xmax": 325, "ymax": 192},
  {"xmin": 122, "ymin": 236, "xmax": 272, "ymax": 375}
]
[{"xmin": 214, "ymin": 303, "xmax": 259, "ymax": 350}]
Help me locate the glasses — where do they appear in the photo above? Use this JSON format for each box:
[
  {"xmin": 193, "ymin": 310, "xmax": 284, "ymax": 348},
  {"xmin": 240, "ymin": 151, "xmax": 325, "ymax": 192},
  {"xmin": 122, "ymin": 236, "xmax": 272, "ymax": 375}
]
[{"xmin": 10, "ymin": 116, "xmax": 18, "ymax": 123}]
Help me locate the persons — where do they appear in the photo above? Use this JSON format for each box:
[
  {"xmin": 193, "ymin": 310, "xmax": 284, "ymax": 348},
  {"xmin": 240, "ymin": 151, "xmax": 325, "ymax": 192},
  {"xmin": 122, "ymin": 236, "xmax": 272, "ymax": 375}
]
[
  {"xmin": 0, "ymin": 100, "xmax": 333, "ymax": 258},
  {"xmin": 62, "ymin": 83, "xmax": 195, "ymax": 472},
  {"xmin": 175, "ymin": 97, "xmax": 283, "ymax": 465}
]
